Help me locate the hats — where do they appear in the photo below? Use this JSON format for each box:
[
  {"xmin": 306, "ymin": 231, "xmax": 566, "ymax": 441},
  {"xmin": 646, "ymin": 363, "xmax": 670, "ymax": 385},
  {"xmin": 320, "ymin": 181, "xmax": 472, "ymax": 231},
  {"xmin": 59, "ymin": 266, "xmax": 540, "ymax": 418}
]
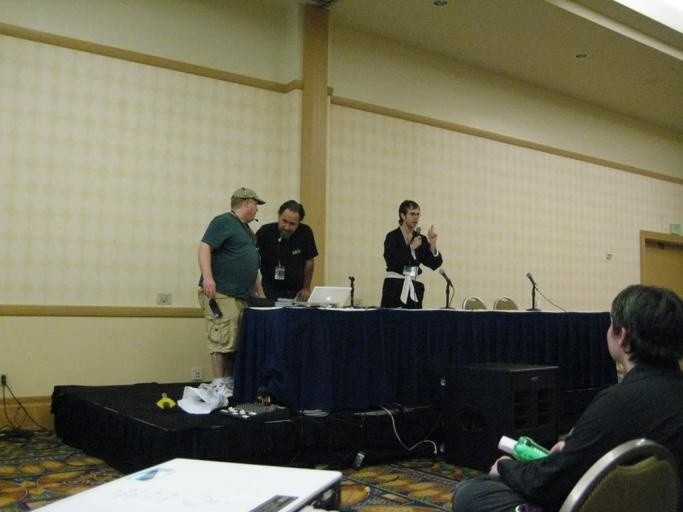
[{"xmin": 231, "ymin": 188, "xmax": 266, "ymax": 204}]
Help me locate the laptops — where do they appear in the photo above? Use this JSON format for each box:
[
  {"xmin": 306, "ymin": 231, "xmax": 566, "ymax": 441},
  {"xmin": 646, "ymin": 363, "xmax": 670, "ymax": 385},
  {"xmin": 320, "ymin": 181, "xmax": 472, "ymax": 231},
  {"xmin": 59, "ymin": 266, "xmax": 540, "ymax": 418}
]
[{"xmin": 295, "ymin": 286, "xmax": 352, "ymax": 307}]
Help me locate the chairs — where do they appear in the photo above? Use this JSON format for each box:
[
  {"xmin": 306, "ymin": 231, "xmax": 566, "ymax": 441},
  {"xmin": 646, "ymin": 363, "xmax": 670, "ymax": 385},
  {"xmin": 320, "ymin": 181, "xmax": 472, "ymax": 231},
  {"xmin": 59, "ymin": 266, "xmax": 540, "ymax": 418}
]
[
  {"xmin": 461, "ymin": 295, "xmax": 488, "ymax": 309},
  {"xmin": 493, "ymin": 297, "xmax": 517, "ymax": 309},
  {"xmin": 558, "ymin": 438, "xmax": 682, "ymax": 512}
]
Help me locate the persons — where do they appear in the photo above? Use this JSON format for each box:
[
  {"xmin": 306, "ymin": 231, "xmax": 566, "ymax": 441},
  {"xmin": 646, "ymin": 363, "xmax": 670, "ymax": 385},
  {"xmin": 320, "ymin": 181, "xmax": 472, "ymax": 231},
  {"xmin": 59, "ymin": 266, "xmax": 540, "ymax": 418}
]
[
  {"xmin": 449, "ymin": 284, "xmax": 682, "ymax": 511},
  {"xmin": 196, "ymin": 187, "xmax": 266, "ymax": 397},
  {"xmin": 252, "ymin": 200, "xmax": 319, "ymax": 302},
  {"xmin": 379, "ymin": 200, "xmax": 443, "ymax": 310}
]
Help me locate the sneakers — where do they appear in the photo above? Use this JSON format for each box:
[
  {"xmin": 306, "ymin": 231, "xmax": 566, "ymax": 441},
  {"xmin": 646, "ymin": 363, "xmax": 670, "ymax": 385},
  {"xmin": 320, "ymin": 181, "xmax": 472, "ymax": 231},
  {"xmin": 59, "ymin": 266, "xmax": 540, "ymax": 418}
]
[{"xmin": 214, "ymin": 381, "xmax": 234, "ymax": 397}]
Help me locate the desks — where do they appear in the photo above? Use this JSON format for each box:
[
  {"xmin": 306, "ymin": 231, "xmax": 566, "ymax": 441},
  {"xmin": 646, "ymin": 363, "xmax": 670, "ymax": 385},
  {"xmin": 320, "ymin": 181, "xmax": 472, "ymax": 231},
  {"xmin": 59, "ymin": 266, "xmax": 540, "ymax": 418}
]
[{"xmin": 232, "ymin": 308, "xmax": 618, "ymax": 414}]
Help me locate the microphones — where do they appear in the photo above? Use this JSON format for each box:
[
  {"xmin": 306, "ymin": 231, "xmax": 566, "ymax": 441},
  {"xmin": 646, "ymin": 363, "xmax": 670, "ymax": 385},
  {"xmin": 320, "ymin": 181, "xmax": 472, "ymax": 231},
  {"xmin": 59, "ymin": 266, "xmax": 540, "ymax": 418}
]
[
  {"xmin": 526, "ymin": 273, "xmax": 537, "ymax": 289},
  {"xmin": 254, "ymin": 217, "xmax": 258, "ymax": 221},
  {"xmin": 439, "ymin": 269, "xmax": 453, "ymax": 287},
  {"xmin": 416, "ymin": 227, "xmax": 421, "ymax": 236}
]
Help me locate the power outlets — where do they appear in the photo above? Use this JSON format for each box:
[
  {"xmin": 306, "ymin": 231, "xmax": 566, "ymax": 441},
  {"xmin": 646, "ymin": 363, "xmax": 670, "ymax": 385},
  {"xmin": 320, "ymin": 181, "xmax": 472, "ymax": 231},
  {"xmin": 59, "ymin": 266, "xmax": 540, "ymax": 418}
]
[{"xmin": 191, "ymin": 367, "xmax": 204, "ymax": 380}]
